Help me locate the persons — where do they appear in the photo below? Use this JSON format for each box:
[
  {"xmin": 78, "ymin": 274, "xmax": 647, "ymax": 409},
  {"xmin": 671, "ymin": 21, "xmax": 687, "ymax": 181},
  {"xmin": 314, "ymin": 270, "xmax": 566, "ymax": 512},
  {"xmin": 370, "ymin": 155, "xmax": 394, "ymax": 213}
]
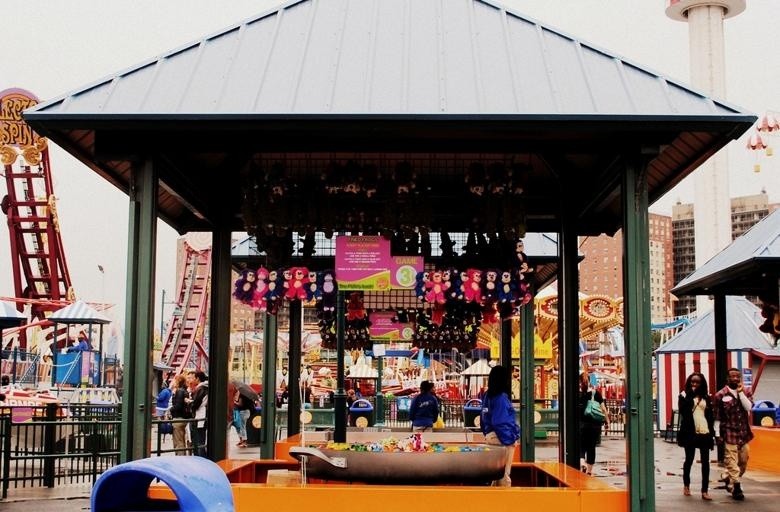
[
  {"xmin": 713, "ymin": 367, "xmax": 754, "ymax": 500},
  {"xmin": 409, "ymin": 380, "xmax": 439, "ymax": 432},
  {"xmin": 67, "ymin": 333, "xmax": 88, "ymax": 353},
  {"xmin": 675, "ymin": 370, "xmax": 718, "ymax": 503},
  {"xmin": 478, "ymin": 365, "xmax": 522, "ymax": 486},
  {"xmin": 151, "ymin": 368, "xmax": 376, "ymax": 456},
  {"xmin": 579, "ymin": 371, "xmax": 611, "ymax": 476},
  {"xmin": 429, "ymin": 382, "xmax": 442, "ymax": 404}
]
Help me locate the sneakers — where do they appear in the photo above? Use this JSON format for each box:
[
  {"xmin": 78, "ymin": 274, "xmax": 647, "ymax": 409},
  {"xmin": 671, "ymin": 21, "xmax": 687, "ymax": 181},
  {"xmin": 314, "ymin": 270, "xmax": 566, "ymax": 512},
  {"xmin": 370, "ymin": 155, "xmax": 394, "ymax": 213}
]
[
  {"xmin": 684, "ymin": 484, "xmax": 689, "ymax": 495},
  {"xmin": 702, "ymin": 492, "xmax": 713, "ymax": 500}
]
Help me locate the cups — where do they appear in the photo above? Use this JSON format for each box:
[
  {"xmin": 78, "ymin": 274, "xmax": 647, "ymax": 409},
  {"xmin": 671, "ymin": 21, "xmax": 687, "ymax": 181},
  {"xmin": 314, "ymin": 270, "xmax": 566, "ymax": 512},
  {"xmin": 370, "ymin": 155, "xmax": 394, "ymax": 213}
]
[{"xmin": 722, "ymin": 394, "xmax": 734, "ymax": 409}]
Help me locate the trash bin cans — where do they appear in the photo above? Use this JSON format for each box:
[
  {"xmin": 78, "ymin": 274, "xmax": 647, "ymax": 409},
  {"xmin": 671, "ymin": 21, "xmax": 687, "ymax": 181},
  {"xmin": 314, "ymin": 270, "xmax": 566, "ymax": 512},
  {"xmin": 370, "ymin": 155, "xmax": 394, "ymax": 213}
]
[
  {"xmin": 751, "ymin": 400, "xmax": 777, "ymax": 426},
  {"xmin": 463, "ymin": 399, "xmax": 483, "ymax": 427},
  {"xmin": 245, "ymin": 407, "xmax": 264, "ymax": 444},
  {"xmin": 349, "ymin": 399, "xmax": 374, "ymax": 427},
  {"xmin": 90, "ymin": 456, "xmax": 236, "ymax": 512}
]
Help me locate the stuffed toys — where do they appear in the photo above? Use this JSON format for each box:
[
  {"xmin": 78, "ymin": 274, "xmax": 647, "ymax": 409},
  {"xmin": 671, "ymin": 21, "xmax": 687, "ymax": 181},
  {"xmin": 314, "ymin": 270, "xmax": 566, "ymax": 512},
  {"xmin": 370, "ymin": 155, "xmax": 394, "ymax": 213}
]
[{"xmin": 232, "ymin": 159, "xmax": 531, "ymax": 352}]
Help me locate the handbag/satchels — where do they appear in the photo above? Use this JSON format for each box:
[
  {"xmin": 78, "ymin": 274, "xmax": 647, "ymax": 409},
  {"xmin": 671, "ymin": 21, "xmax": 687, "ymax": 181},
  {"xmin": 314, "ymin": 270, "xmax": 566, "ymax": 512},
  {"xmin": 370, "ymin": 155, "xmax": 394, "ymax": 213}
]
[
  {"xmin": 160, "ymin": 423, "xmax": 173, "ymax": 434},
  {"xmin": 583, "ymin": 400, "xmax": 605, "ymax": 421},
  {"xmin": 677, "ymin": 429, "xmax": 693, "ymax": 447}
]
